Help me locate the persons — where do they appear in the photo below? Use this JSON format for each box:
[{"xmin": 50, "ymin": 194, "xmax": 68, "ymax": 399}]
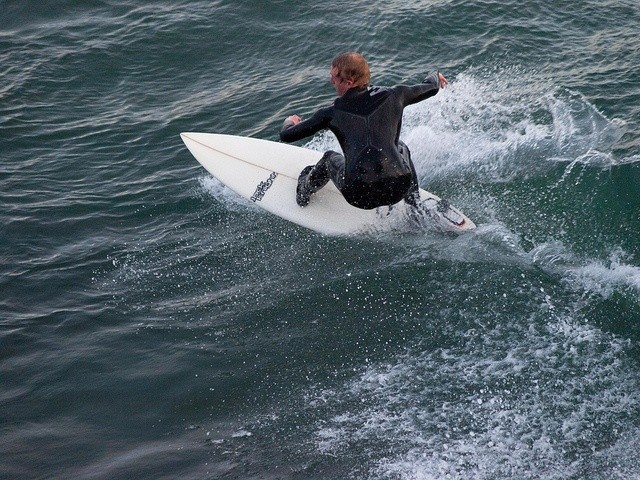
[{"xmin": 279, "ymin": 52, "xmax": 449, "ymax": 218}]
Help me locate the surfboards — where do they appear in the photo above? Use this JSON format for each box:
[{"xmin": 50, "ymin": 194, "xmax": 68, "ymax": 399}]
[{"xmin": 180, "ymin": 131, "xmax": 477, "ymax": 240}]
